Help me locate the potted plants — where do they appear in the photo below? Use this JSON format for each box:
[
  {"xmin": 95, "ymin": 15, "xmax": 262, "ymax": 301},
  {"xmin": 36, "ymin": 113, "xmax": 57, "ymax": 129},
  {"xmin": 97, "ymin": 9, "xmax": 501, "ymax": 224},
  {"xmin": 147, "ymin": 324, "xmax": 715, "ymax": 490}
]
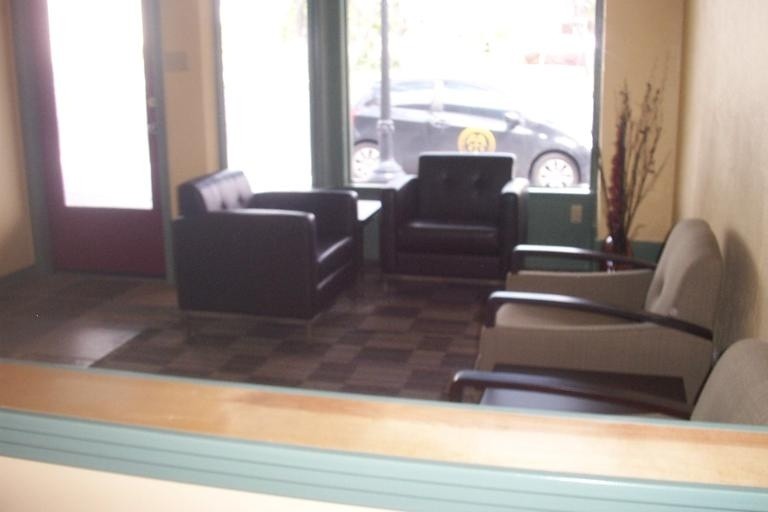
[{"xmin": 589, "ymin": 77, "xmax": 672, "ymax": 271}]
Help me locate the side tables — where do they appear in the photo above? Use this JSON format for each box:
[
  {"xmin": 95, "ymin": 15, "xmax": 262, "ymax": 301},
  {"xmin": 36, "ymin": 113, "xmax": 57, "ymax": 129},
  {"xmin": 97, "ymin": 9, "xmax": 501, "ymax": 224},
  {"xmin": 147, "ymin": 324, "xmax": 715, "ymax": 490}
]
[
  {"xmin": 354, "ymin": 199, "xmax": 383, "ymax": 265},
  {"xmin": 479, "ymin": 362, "xmax": 691, "ymax": 420}
]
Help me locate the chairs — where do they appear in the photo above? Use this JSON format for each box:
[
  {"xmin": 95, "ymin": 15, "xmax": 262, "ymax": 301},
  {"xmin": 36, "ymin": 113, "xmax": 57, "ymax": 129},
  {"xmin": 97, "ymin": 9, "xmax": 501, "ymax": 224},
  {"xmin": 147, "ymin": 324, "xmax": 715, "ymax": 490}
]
[
  {"xmin": 173, "ymin": 168, "xmax": 358, "ymax": 319},
  {"xmin": 448, "ymin": 336, "xmax": 767, "ymax": 428},
  {"xmin": 382, "ymin": 151, "xmax": 529, "ymax": 278},
  {"xmin": 479, "ymin": 217, "xmax": 722, "ymax": 403}
]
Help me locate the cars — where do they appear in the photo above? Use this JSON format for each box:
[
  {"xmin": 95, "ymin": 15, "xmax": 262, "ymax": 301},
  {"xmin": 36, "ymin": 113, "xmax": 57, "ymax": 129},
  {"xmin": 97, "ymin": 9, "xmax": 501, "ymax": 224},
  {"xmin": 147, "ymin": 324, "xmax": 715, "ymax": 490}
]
[{"xmin": 349, "ymin": 88, "xmax": 591, "ymax": 189}]
